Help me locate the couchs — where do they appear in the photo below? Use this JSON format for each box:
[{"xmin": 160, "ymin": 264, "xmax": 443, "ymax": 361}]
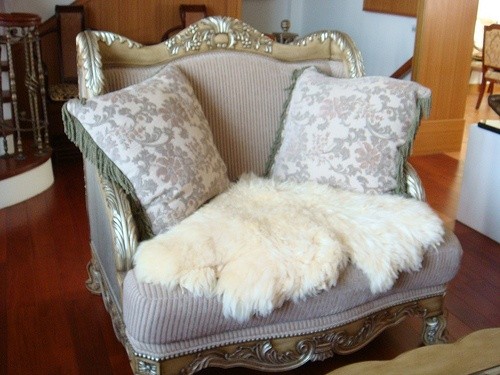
[{"xmin": 59, "ymin": 11, "xmax": 465, "ymax": 374}]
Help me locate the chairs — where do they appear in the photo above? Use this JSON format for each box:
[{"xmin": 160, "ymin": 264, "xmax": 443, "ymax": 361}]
[{"xmin": 475, "ymin": 24, "xmax": 499, "ymax": 109}]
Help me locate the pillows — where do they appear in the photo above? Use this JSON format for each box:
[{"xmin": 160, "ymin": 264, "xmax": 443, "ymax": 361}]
[
  {"xmin": 265, "ymin": 65, "xmax": 432, "ymax": 199},
  {"xmin": 61, "ymin": 62, "xmax": 232, "ymax": 242}
]
